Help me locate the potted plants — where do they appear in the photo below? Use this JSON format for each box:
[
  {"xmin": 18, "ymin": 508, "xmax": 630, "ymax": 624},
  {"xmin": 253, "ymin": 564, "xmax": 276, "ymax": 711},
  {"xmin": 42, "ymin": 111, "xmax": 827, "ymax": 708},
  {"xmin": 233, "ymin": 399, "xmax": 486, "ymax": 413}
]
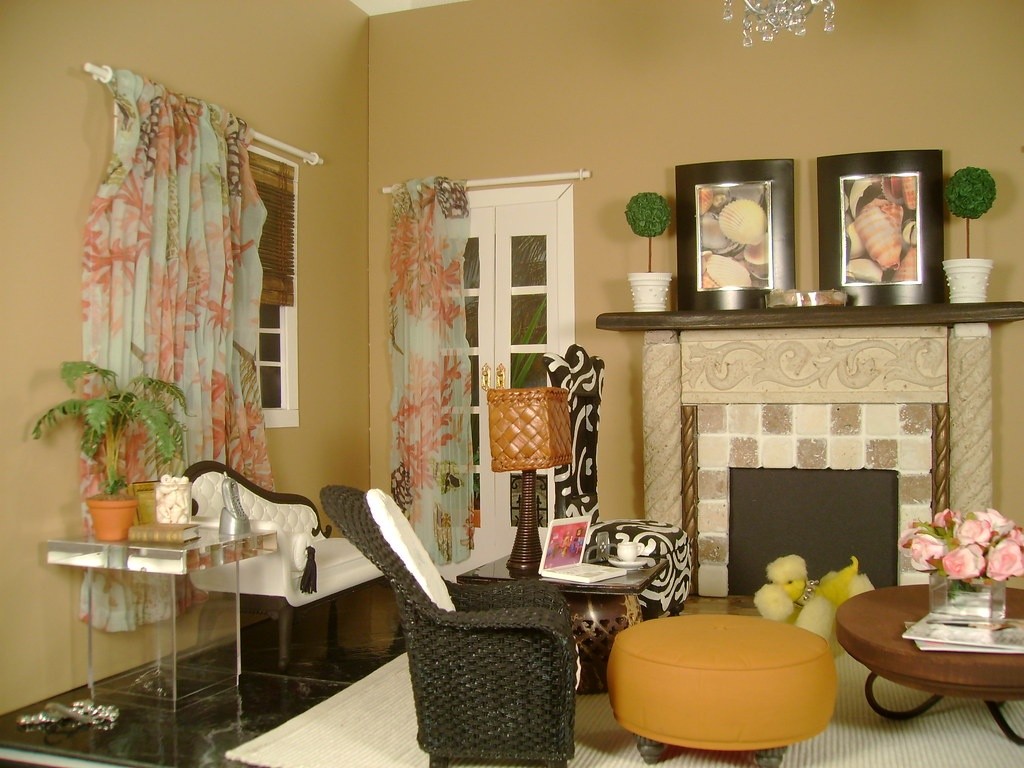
[
  {"xmin": 35, "ymin": 361, "xmax": 195, "ymax": 541},
  {"xmin": 942, "ymin": 168, "xmax": 998, "ymax": 302},
  {"xmin": 624, "ymin": 189, "xmax": 673, "ymax": 311}
]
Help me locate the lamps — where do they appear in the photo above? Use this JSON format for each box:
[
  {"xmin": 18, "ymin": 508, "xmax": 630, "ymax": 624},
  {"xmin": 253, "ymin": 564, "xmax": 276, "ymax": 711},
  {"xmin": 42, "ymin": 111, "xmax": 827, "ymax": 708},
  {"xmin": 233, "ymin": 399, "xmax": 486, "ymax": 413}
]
[
  {"xmin": 724, "ymin": 0, "xmax": 833, "ymax": 47},
  {"xmin": 490, "ymin": 386, "xmax": 573, "ymax": 571}
]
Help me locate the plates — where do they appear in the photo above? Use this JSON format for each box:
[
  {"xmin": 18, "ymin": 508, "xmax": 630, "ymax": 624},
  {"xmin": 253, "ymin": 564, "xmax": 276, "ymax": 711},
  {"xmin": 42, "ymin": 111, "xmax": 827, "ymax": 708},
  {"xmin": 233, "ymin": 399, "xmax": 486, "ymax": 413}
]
[{"xmin": 608, "ymin": 558, "xmax": 646, "ymax": 571}]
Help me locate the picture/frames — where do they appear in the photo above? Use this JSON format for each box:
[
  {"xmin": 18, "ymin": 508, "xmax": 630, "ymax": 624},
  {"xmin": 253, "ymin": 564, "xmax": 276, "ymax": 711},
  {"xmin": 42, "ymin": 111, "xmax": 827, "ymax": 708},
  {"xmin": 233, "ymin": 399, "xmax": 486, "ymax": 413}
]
[
  {"xmin": 818, "ymin": 149, "xmax": 952, "ymax": 306},
  {"xmin": 675, "ymin": 158, "xmax": 797, "ymax": 308}
]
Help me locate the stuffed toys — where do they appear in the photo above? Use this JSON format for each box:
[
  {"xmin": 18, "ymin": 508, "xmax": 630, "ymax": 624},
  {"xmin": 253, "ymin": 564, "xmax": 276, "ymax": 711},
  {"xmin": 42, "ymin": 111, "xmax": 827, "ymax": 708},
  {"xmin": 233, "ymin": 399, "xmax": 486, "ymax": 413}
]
[{"xmin": 754, "ymin": 554, "xmax": 875, "ymax": 641}]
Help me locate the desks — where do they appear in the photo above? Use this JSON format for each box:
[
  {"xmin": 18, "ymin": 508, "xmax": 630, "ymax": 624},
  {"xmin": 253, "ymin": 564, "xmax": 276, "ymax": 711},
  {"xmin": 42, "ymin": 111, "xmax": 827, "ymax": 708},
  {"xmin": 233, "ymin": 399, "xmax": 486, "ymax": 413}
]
[
  {"xmin": 44, "ymin": 526, "xmax": 277, "ymax": 716},
  {"xmin": 837, "ymin": 584, "xmax": 1024, "ymax": 742},
  {"xmin": 610, "ymin": 614, "xmax": 838, "ymax": 767},
  {"xmin": 459, "ymin": 557, "xmax": 668, "ymax": 691}
]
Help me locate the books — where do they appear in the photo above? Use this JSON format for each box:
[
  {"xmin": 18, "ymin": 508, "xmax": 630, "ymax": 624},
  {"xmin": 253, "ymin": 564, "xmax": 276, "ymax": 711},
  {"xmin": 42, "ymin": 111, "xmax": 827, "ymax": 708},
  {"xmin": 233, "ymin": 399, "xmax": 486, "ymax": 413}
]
[
  {"xmin": 127, "ymin": 522, "xmax": 201, "ymax": 544},
  {"xmin": 902, "ymin": 612, "xmax": 1024, "ymax": 653}
]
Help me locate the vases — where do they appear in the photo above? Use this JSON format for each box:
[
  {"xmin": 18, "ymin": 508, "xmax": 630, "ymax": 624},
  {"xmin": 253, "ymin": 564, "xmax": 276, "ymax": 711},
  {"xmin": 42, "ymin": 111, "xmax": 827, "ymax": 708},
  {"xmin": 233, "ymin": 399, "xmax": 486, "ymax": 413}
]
[{"xmin": 929, "ymin": 569, "xmax": 1007, "ymax": 621}]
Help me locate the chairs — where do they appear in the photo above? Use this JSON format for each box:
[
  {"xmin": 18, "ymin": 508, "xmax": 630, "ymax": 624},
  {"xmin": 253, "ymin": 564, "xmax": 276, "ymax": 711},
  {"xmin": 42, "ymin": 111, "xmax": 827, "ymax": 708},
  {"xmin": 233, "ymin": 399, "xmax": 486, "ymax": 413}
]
[
  {"xmin": 179, "ymin": 456, "xmax": 395, "ymax": 671},
  {"xmin": 543, "ymin": 344, "xmax": 693, "ymax": 619},
  {"xmin": 320, "ymin": 483, "xmax": 582, "ymax": 767}
]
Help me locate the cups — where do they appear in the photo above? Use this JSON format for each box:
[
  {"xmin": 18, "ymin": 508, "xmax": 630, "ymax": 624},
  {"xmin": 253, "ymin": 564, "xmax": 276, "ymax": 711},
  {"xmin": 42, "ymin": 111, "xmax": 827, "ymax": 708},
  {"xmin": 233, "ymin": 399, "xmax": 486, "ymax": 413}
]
[{"xmin": 617, "ymin": 542, "xmax": 645, "ymax": 562}]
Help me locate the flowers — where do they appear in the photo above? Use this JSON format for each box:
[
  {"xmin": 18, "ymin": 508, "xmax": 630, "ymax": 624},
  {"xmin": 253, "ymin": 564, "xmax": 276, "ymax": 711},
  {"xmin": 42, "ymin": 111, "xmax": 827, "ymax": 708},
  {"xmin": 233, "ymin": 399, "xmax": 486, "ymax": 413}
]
[{"xmin": 893, "ymin": 474, "xmax": 1024, "ymax": 583}]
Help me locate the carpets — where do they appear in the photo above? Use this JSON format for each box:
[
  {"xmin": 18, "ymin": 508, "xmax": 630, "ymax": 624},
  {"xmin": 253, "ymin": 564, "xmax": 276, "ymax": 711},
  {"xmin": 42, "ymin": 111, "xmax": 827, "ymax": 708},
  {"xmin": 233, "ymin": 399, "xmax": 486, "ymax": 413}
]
[{"xmin": 225, "ymin": 615, "xmax": 1024, "ymax": 767}]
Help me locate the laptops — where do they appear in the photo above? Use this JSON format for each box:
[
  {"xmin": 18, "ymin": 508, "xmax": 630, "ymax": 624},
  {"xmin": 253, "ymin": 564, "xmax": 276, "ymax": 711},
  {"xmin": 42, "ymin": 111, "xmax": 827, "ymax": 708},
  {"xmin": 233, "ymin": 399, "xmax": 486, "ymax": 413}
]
[{"xmin": 538, "ymin": 515, "xmax": 628, "ymax": 584}]
